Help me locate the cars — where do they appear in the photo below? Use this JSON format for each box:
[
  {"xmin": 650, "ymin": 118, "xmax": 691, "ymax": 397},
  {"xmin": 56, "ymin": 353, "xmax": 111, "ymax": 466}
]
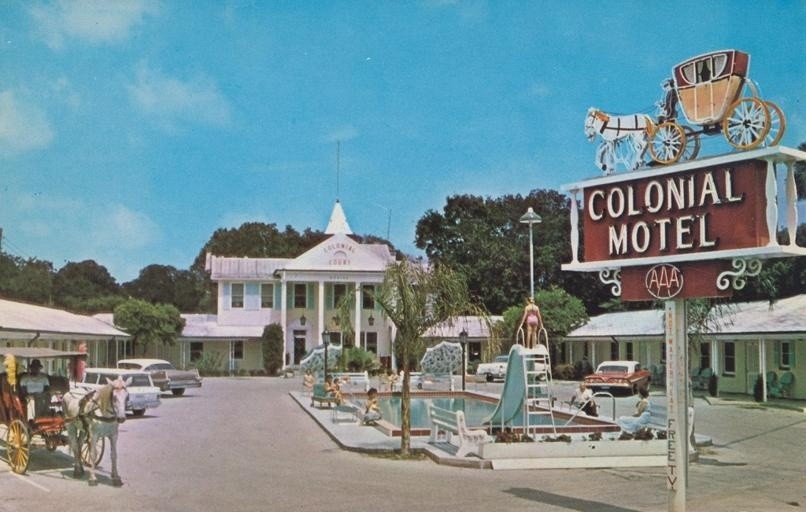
[
  {"xmin": 85, "ymin": 357, "xmax": 202, "ymax": 416},
  {"xmin": 476, "ymin": 353, "xmax": 550, "ymax": 381},
  {"xmin": 584, "ymin": 360, "xmax": 652, "ymax": 396}
]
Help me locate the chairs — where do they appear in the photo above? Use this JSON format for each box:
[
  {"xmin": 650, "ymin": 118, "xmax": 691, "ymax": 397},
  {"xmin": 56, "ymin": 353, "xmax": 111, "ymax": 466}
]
[
  {"xmin": 648, "ymin": 365, "xmax": 713, "ymax": 391},
  {"xmin": 0, "ymin": 371, "xmax": 70, "ymax": 426},
  {"xmin": 775, "ymin": 372, "xmax": 794, "ymax": 400},
  {"xmin": 766, "ymin": 371, "xmax": 776, "ymax": 401}
]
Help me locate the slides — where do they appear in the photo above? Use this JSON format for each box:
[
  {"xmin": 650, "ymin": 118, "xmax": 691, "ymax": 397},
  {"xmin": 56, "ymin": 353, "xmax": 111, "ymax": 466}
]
[{"xmin": 479, "ymin": 344, "xmax": 524, "ymax": 426}]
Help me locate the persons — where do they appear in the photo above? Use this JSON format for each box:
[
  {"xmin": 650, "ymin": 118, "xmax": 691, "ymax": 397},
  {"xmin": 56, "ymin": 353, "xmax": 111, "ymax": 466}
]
[
  {"xmin": 611, "ymin": 387, "xmax": 651, "ymax": 440},
  {"xmin": 301, "ymin": 367, "xmax": 316, "ymax": 396},
  {"xmin": 655, "ymin": 79, "xmax": 678, "ymax": 145},
  {"xmin": 568, "ymin": 380, "xmax": 598, "ymax": 416},
  {"xmin": 361, "ymin": 388, "xmax": 382, "ymax": 426},
  {"xmin": 330, "ymin": 377, "xmax": 343, "ymax": 400},
  {"xmin": 519, "ymin": 295, "xmax": 545, "ymax": 349},
  {"xmin": 324, "ymin": 373, "xmax": 346, "ymax": 404},
  {"xmin": 18, "ymin": 359, "xmax": 62, "ymax": 417},
  {"xmin": 387, "ymin": 368, "xmax": 398, "ymax": 392}
]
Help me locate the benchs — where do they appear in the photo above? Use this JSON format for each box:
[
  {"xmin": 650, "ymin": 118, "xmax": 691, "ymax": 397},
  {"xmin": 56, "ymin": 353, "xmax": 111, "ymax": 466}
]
[
  {"xmin": 311, "ymin": 384, "xmax": 339, "ymax": 409},
  {"xmin": 391, "ymin": 371, "xmax": 425, "ymax": 393},
  {"xmin": 423, "ymin": 398, "xmax": 496, "ymax": 459},
  {"xmin": 638, "ymin": 400, "xmax": 667, "ymax": 426},
  {"xmin": 328, "ymin": 370, "xmax": 369, "ymax": 394}
]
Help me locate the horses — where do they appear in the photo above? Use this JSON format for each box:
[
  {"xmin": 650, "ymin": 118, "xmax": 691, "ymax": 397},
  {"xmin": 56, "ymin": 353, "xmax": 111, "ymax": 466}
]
[{"xmin": 62, "ymin": 375, "xmax": 133, "ymax": 486}]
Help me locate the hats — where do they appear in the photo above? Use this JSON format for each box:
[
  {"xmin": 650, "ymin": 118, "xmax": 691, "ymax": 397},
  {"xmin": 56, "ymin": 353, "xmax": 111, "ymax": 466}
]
[{"xmin": 29, "ymin": 359, "xmax": 43, "ymax": 369}]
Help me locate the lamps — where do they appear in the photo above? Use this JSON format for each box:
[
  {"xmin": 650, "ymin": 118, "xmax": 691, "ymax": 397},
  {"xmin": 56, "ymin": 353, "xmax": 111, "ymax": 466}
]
[
  {"xmin": 300, "ymin": 307, "xmax": 306, "ymax": 325},
  {"xmin": 368, "ymin": 299, "xmax": 375, "ymax": 325},
  {"xmin": 335, "ymin": 311, "xmax": 341, "ymax": 325}
]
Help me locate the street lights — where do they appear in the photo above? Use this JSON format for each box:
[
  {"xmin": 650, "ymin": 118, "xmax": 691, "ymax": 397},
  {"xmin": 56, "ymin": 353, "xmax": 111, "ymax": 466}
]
[
  {"xmin": 460, "ymin": 328, "xmax": 468, "ymax": 389},
  {"xmin": 519, "ymin": 204, "xmax": 543, "ymax": 302},
  {"xmin": 321, "ymin": 323, "xmax": 331, "ymax": 375}
]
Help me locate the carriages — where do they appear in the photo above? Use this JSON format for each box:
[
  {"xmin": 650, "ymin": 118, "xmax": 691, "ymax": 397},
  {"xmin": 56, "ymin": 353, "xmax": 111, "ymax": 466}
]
[
  {"xmin": 586, "ymin": 49, "xmax": 786, "ymax": 177},
  {"xmin": 0, "ymin": 347, "xmax": 133, "ymax": 487}
]
